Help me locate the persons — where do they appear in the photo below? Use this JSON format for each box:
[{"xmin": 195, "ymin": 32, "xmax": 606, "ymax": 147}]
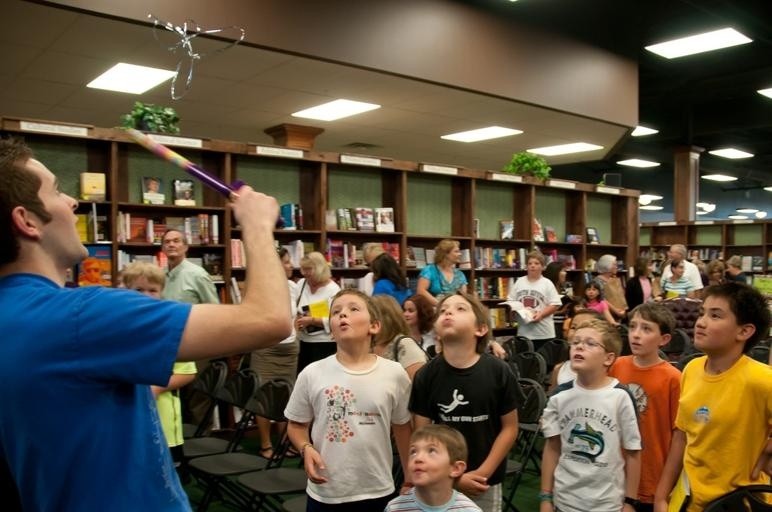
[
  {"xmin": 409, "ymin": 291, "xmax": 519, "ymax": 498},
  {"xmin": 552, "ymin": 308, "xmax": 603, "ymax": 390},
  {"xmin": 252, "ymin": 246, "xmax": 300, "ymax": 460},
  {"xmin": 162, "ymin": 229, "xmax": 219, "ymax": 304},
  {"xmin": 653, "ymin": 282, "xmax": 771, "ymax": 512},
  {"xmin": 662, "ymin": 259, "xmax": 694, "ymax": 296},
  {"xmin": 386, "ymin": 425, "xmax": 486, "ymax": 511},
  {"xmin": 545, "ymin": 261, "xmax": 573, "ymax": 318},
  {"xmin": 581, "ymin": 281, "xmax": 620, "ymax": 325},
  {"xmin": 608, "ymin": 304, "xmax": 683, "ymax": 512},
  {"xmin": 144, "ymin": 180, "xmax": 165, "ymax": 205},
  {"xmin": 591, "ymin": 255, "xmax": 628, "ymax": 315},
  {"xmin": 540, "ymin": 319, "xmax": 642, "ymax": 512},
  {"xmin": 181, "ymin": 191, "xmax": 192, "ymax": 200},
  {"xmin": 706, "ymin": 259, "xmax": 724, "ymax": 285},
  {"xmin": 375, "ymin": 212, "xmax": 393, "ymax": 231},
  {"xmin": 80, "ymin": 257, "xmax": 111, "ymax": 286},
  {"xmin": 123, "ymin": 262, "xmax": 197, "ymax": 465},
  {"xmin": 284, "ymin": 289, "xmax": 415, "ymax": 511},
  {"xmin": 371, "ymin": 253, "xmax": 412, "ymax": 304},
  {"xmin": 726, "ymin": 256, "xmax": 747, "ymax": 283},
  {"xmin": 404, "ymin": 297, "xmax": 431, "ymax": 340},
  {"xmin": 626, "ymin": 258, "xmax": 652, "ymax": 310},
  {"xmin": 418, "ymin": 241, "xmax": 468, "ymax": 304},
  {"xmin": 660, "ymin": 244, "xmax": 704, "ymax": 292},
  {"xmin": 0, "ymin": 139, "xmax": 292, "ymax": 512},
  {"xmin": 371, "ymin": 293, "xmax": 425, "ymax": 376},
  {"xmin": 297, "ymin": 251, "xmax": 341, "ymax": 377},
  {"xmin": 507, "ymin": 252, "xmax": 563, "ymax": 350}
]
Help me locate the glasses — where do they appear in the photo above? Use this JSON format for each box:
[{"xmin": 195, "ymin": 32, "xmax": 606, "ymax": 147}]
[{"xmin": 569, "ymin": 338, "xmax": 609, "ymax": 353}]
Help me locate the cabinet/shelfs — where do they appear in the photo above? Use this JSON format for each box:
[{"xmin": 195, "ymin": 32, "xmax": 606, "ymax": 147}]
[
  {"xmin": 2, "ymin": 118, "xmax": 639, "ymax": 331},
  {"xmin": 637, "ymin": 221, "xmax": 772, "ymax": 299}
]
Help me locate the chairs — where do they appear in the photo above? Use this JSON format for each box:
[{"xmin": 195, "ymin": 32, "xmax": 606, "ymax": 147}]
[
  {"xmin": 503, "ymin": 379, "xmax": 550, "ymax": 512},
  {"xmin": 237, "ymin": 465, "xmax": 308, "ymax": 511},
  {"xmin": 178, "ymin": 370, "xmax": 257, "ymax": 512},
  {"xmin": 536, "ymin": 337, "xmax": 573, "ymax": 368},
  {"xmin": 501, "ymin": 336, "xmax": 530, "ymax": 355},
  {"xmin": 167, "ymin": 361, "xmax": 228, "ymax": 486},
  {"xmin": 504, "ymin": 352, "xmax": 546, "ymax": 385},
  {"xmin": 192, "ymin": 382, "xmax": 289, "ymax": 510}
]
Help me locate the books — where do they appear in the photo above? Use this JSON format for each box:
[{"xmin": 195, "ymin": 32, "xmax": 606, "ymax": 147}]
[
  {"xmin": 117, "ymin": 211, "xmax": 218, "ymax": 246},
  {"xmin": 173, "ymin": 178, "xmax": 196, "ymax": 205},
  {"xmin": 475, "ymin": 277, "xmax": 518, "ymax": 298},
  {"xmin": 327, "ymin": 239, "xmax": 356, "ymax": 267},
  {"xmin": 477, "ymin": 248, "xmax": 527, "ymax": 270},
  {"xmin": 327, "ymin": 206, "xmax": 394, "ymax": 231},
  {"xmin": 141, "ymin": 176, "xmax": 165, "ymax": 205}
]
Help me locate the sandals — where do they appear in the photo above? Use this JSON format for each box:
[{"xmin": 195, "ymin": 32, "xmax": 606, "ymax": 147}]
[{"xmin": 257, "ymin": 446, "xmax": 279, "ymax": 460}]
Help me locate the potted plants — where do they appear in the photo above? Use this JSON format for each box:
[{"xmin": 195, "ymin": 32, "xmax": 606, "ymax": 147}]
[
  {"xmin": 122, "ymin": 102, "xmax": 182, "ymax": 136},
  {"xmin": 504, "ymin": 150, "xmax": 551, "ymax": 179}
]
